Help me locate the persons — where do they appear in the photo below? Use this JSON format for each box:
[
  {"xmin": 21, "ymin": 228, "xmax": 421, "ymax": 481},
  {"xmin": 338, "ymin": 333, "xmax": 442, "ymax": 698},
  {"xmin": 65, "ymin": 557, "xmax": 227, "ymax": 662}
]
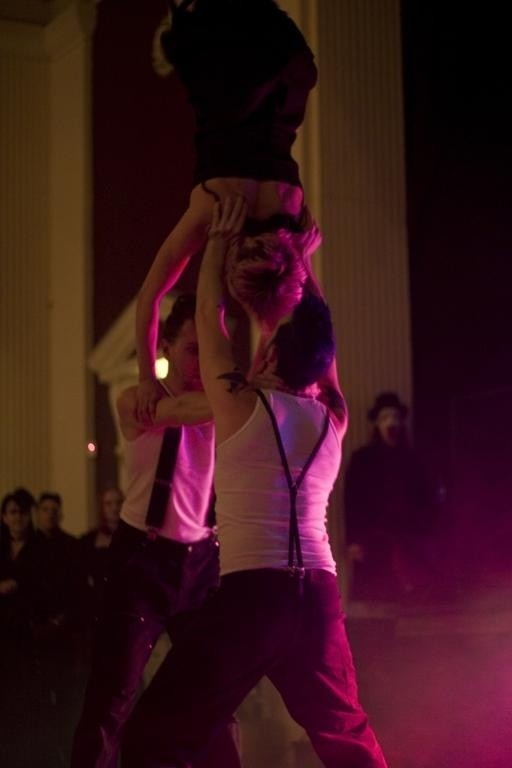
[
  {"xmin": 115, "ymin": 189, "xmax": 393, "ymax": 767},
  {"xmin": 341, "ymin": 386, "xmax": 413, "ymax": 593},
  {"xmin": 2, "ymin": 482, "xmax": 509, "ymax": 766},
  {"xmin": 131, "ymin": 0, "xmax": 324, "ymax": 434},
  {"xmin": 75, "ymin": 283, "xmax": 270, "ymax": 764}
]
